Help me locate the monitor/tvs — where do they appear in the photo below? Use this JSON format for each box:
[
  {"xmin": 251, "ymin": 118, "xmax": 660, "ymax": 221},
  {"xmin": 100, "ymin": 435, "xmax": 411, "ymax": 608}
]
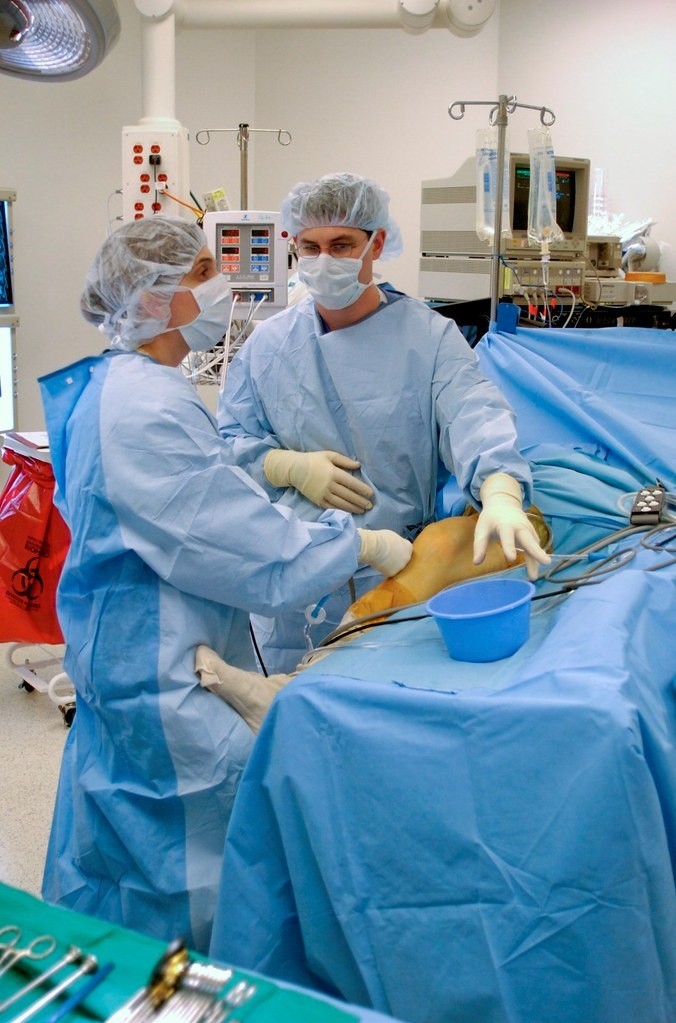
[{"xmin": 417, "ymin": 148, "xmax": 588, "ymax": 253}]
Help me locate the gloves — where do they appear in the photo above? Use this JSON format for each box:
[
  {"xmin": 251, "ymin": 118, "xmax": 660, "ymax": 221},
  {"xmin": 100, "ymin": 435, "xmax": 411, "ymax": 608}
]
[
  {"xmin": 473, "ymin": 473, "xmax": 551, "ymax": 580},
  {"xmin": 356, "ymin": 527, "xmax": 414, "ymax": 578},
  {"xmin": 263, "ymin": 448, "xmax": 374, "ymax": 515}
]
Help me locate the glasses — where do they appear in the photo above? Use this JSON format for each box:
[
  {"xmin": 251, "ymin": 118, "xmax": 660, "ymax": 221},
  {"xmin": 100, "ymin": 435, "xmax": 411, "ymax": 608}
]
[{"xmin": 294, "ymin": 235, "xmax": 368, "ymax": 259}]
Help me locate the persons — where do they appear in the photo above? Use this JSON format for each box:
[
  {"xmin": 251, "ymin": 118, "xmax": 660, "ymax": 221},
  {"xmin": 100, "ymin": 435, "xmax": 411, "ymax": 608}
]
[
  {"xmin": 36, "ymin": 219, "xmax": 414, "ymax": 957},
  {"xmin": 217, "ymin": 172, "xmax": 552, "ymax": 580},
  {"xmin": 198, "ymin": 510, "xmax": 547, "ymax": 733}
]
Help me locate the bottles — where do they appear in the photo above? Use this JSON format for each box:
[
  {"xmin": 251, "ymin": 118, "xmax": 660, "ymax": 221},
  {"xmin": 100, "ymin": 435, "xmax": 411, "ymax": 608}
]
[{"xmin": 497, "ymin": 295, "xmax": 517, "ymax": 333}]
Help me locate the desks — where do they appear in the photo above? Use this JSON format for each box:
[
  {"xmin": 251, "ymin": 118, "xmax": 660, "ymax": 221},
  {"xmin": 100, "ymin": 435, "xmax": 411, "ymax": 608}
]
[{"xmin": 0, "ymin": 882, "xmax": 409, "ymax": 1023}]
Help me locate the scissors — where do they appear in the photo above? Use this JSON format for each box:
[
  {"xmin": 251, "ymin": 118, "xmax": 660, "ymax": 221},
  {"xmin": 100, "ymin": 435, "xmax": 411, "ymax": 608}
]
[
  {"xmin": 104, "ymin": 937, "xmax": 257, "ymax": 1022},
  {"xmin": 0, "ymin": 925, "xmax": 56, "ymax": 977}
]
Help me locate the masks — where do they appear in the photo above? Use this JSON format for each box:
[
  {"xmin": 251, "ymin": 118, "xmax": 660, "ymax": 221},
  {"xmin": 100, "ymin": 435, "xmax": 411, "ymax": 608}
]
[
  {"xmin": 296, "ymin": 229, "xmax": 379, "ymax": 311},
  {"xmin": 109, "ymin": 274, "xmax": 234, "ymax": 353}
]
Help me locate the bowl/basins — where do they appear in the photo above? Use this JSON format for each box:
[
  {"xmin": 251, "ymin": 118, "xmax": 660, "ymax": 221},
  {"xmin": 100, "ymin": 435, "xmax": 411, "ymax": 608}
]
[{"xmin": 424, "ymin": 577, "xmax": 537, "ymax": 665}]
